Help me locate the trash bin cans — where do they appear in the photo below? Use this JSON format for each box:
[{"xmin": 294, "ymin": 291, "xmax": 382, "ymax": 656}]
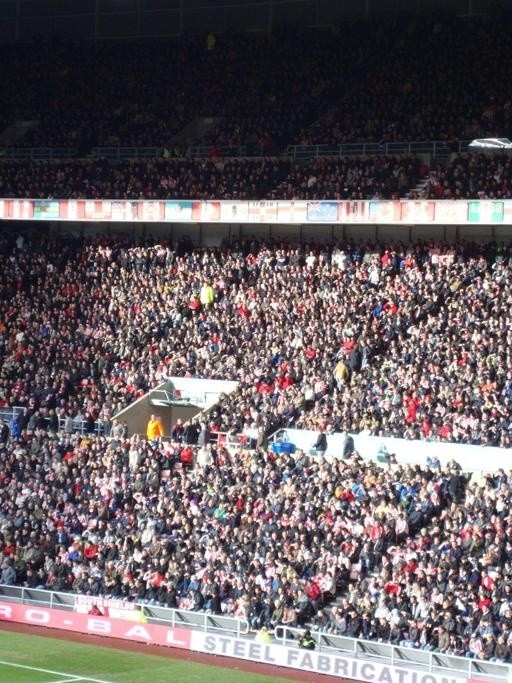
[{"xmin": 273, "ymin": 442, "xmax": 296, "ymax": 453}]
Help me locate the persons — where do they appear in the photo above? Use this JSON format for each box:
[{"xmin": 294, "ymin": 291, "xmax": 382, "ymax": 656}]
[{"xmin": 0, "ymin": 0, "xmax": 512, "ymax": 668}]
